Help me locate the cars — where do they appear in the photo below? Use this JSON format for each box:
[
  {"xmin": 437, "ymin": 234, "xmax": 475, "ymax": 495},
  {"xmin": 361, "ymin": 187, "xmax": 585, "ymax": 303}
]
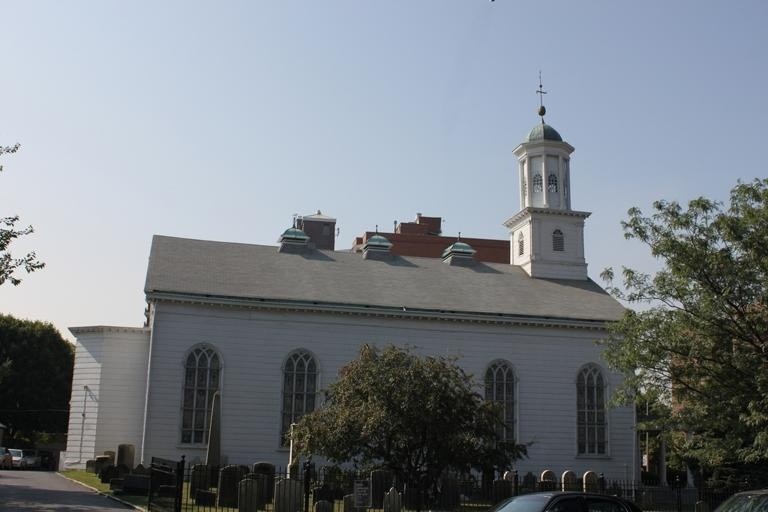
[
  {"xmin": 486, "ymin": 491, "xmax": 645, "ymax": 512},
  {"xmin": 0, "ymin": 446, "xmax": 57, "ymax": 472},
  {"xmin": 711, "ymin": 489, "xmax": 767, "ymax": 512}
]
